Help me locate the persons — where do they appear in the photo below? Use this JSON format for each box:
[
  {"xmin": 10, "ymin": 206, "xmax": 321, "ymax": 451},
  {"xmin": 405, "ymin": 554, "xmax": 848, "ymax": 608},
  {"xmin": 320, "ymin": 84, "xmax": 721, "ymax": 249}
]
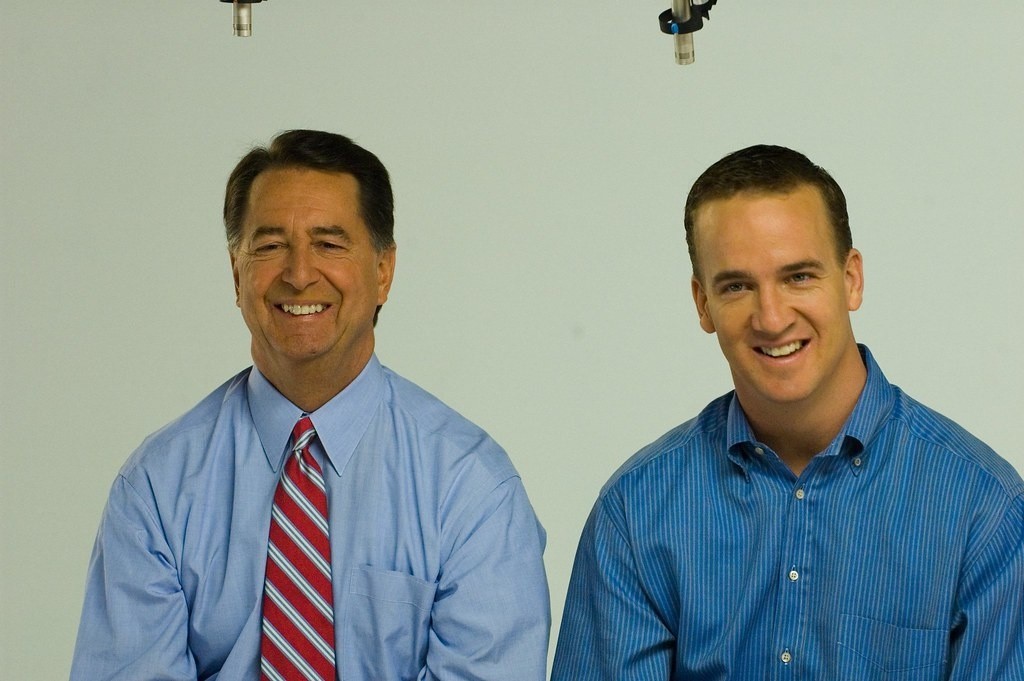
[
  {"xmin": 70, "ymin": 130, "xmax": 549, "ymax": 680},
  {"xmin": 550, "ymin": 144, "xmax": 1024, "ymax": 680}
]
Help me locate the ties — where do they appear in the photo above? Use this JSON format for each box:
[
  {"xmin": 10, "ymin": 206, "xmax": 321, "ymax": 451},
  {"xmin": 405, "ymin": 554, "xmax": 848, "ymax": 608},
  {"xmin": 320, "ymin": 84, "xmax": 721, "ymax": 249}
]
[{"xmin": 261, "ymin": 416, "xmax": 337, "ymax": 681}]
